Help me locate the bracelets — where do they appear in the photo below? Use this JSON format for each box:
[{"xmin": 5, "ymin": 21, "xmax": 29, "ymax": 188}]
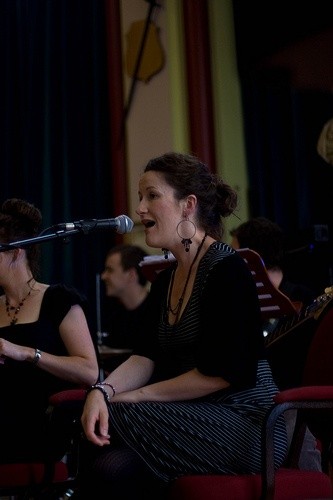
[
  {"xmin": 32, "ymin": 348, "xmax": 41, "ymax": 368},
  {"xmin": 85, "ymin": 382, "xmax": 115, "ymax": 407}
]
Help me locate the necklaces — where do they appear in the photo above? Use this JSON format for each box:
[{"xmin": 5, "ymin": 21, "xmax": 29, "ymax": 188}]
[
  {"xmin": 168, "ymin": 231, "xmax": 208, "ymax": 316},
  {"xmin": 5, "ymin": 282, "xmax": 36, "ymax": 326}
]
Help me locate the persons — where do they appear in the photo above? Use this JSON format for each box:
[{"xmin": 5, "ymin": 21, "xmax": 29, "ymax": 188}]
[
  {"xmin": 86, "ymin": 245, "xmax": 152, "ymax": 375},
  {"xmin": 81, "ymin": 152, "xmax": 288, "ymax": 500},
  {"xmin": 230, "ymin": 217, "xmax": 307, "ymax": 322},
  {"xmin": 0, "ymin": 198, "xmax": 100, "ymax": 465}
]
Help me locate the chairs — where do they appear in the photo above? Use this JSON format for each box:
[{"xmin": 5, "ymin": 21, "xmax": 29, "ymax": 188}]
[
  {"xmin": 0, "ymin": 389, "xmax": 88, "ymax": 500},
  {"xmin": 173, "ymin": 307, "xmax": 333, "ymax": 500}
]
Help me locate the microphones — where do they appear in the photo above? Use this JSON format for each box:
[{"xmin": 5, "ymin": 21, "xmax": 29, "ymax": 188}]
[{"xmin": 57, "ymin": 215, "xmax": 134, "ymax": 234}]
